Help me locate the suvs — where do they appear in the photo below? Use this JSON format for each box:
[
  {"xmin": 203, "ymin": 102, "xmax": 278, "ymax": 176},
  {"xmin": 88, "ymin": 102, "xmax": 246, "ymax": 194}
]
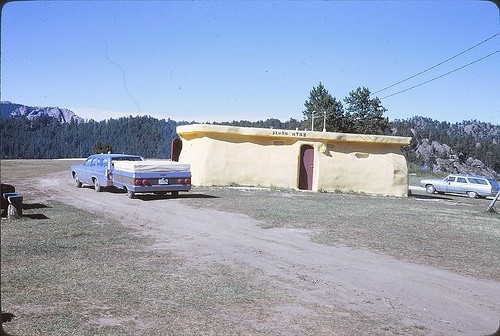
[{"xmin": 420, "ymin": 174, "xmax": 500, "ymax": 198}]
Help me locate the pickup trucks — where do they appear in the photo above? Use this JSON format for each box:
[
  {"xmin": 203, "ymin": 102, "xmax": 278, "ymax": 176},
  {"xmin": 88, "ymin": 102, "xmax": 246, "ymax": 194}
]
[{"xmin": 71, "ymin": 153, "xmax": 191, "ymax": 199}]
